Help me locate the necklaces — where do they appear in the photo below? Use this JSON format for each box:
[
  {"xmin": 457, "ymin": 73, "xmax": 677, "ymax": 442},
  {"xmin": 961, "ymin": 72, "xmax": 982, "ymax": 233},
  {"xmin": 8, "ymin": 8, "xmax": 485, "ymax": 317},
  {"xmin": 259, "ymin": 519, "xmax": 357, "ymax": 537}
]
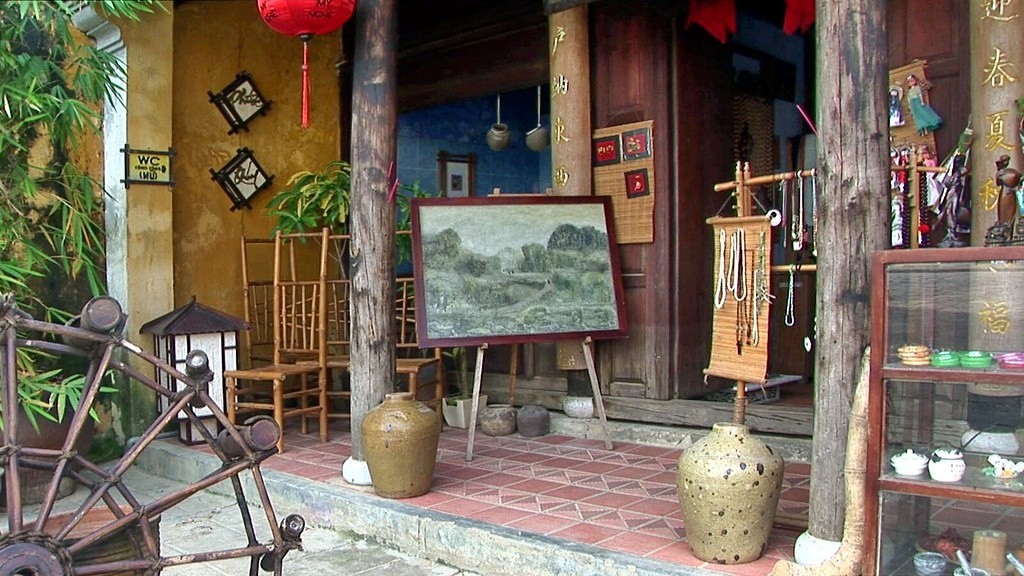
[
  {"xmin": 781, "ymin": 169, "xmax": 817, "ymax": 257},
  {"xmin": 920, "ymin": 165, "xmax": 932, "ymax": 247},
  {"xmin": 714, "ymin": 229, "xmax": 776, "ymax": 355},
  {"xmin": 785, "ymin": 264, "xmax": 811, "ymax": 352},
  {"xmin": 902, "ymin": 162, "xmax": 911, "ymax": 248}
]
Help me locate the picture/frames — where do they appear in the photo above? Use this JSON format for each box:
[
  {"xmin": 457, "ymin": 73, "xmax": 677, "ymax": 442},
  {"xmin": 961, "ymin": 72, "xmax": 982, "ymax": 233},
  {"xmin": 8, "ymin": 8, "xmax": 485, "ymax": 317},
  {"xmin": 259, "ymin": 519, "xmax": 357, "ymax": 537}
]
[
  {"xmin": 624, "ymin": 168, "xmax": 650, "ymax": 198},
  {"xmin": 409, "ymin": 196, "xmax": 632, "ymax": 349},
  {"xmin": 621, "ymin": 128, "xmax": 654, "ymax": 161},
  {"xmin": 593, "ymin": 135, "xmax": 620, "ymax": 167},
  {"xmin": 436, "ymin": 150, "xmax": 477, "ymax": 197}
]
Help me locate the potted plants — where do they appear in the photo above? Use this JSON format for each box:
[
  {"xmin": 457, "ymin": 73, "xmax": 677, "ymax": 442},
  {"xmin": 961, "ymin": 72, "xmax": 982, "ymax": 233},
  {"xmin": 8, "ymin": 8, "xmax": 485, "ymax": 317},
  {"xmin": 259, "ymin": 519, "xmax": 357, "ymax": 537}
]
[
  {"xmin": 0, "ymin": 0, "xmax": 174, "ymax": 506},
  {"xmin": 269, "ymin": 159, "xmax": 489, "ymax": 430}
]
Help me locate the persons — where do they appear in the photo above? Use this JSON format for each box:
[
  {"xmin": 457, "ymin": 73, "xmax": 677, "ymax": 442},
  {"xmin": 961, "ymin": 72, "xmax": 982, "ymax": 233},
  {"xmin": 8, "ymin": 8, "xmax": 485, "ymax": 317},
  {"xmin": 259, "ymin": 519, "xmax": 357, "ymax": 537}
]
[
  {"xmin": 905, "ymin": 74, "xmax": 942, "ymax": 137},
  {"xmin": 995, "ymin": 155, "xmax": 1020, "ymax": 223},
  {"xmin": 892, "ymin": 197, "xmax": 903, "ymax": 247},
  {"xmin": 890, "ymin": 83, "xmax": 905, "ymax": 128},
  {"xmin": 891, "ymin": 143, "xmax": 945, "ymax": 212}
]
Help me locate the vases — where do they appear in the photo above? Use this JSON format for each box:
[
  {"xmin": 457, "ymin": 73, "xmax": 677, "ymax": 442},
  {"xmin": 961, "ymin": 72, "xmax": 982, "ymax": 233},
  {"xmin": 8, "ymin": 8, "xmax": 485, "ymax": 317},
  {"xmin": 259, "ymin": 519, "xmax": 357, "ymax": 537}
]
[
  {"xmin": 360, "ymin": 390, "xmax": 437, "ymax": 499},
  {"xmin": 673, "ymin": 420, "xmax": 785, "ymax": 565}
]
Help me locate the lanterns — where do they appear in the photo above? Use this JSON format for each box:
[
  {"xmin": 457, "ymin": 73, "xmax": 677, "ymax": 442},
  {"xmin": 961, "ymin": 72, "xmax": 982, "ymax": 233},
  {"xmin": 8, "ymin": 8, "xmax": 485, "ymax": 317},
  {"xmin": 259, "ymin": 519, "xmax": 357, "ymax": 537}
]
[{"xmin": 258, "ymin": 0, "xmax": 355, "ymax": 129}]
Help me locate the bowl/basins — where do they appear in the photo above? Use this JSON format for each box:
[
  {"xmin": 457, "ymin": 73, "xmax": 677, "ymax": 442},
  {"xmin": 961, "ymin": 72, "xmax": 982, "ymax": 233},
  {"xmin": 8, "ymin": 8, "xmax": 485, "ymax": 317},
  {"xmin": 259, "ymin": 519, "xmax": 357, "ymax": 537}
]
[{"xmin": 914, "ymin": 552, "xmax": 949, "ymax": 576}]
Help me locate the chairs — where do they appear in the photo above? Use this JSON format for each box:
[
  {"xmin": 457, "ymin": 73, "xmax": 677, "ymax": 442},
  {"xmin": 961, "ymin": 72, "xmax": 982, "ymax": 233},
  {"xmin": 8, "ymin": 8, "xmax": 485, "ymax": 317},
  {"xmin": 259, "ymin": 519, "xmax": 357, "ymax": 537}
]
[{"xmin": 223, "ymin": 225, "xmax": 447, "ymax": 452}]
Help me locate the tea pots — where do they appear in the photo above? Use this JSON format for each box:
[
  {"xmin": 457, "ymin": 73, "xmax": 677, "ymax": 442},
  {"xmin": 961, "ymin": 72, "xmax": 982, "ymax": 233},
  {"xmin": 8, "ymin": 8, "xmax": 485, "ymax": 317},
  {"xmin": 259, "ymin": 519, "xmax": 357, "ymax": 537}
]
[{"xmin": 928, "ymin": 440, "xmax": 966, "ymax": 482}]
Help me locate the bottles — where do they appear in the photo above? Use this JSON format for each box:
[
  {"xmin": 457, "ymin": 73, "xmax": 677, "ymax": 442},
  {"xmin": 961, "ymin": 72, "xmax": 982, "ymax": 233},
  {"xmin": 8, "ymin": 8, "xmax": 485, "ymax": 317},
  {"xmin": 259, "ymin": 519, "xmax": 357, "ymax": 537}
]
[
  {"xmin": 361, "ymin": 393, "xmax": 440, "ymax": 498},
  {"xmin": 479, "ymin": 404, "xmax": 516, "ymax": 436},
  {"xmin": 517, "ymin": 400, "xmax": 549, "ymax": 437},
  {"xmin": 526, "ymin": 127, "xmax": 550, "ymax": 152},
  {"xmin": 487, "ymin": 123, "xmax": 509, "ymax": 151},
  {"xmin": 676, "ymin": 423, "xmax": 785, "ymax": 564}
]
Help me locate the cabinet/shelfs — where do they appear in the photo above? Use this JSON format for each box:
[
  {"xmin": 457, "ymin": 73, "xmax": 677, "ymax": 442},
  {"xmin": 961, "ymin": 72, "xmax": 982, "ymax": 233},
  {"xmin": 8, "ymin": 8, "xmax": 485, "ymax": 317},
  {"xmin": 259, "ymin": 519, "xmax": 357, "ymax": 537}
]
[{"xmin": 861, "ymin": 243, "xmax": 1024, "ymax": 576}]
